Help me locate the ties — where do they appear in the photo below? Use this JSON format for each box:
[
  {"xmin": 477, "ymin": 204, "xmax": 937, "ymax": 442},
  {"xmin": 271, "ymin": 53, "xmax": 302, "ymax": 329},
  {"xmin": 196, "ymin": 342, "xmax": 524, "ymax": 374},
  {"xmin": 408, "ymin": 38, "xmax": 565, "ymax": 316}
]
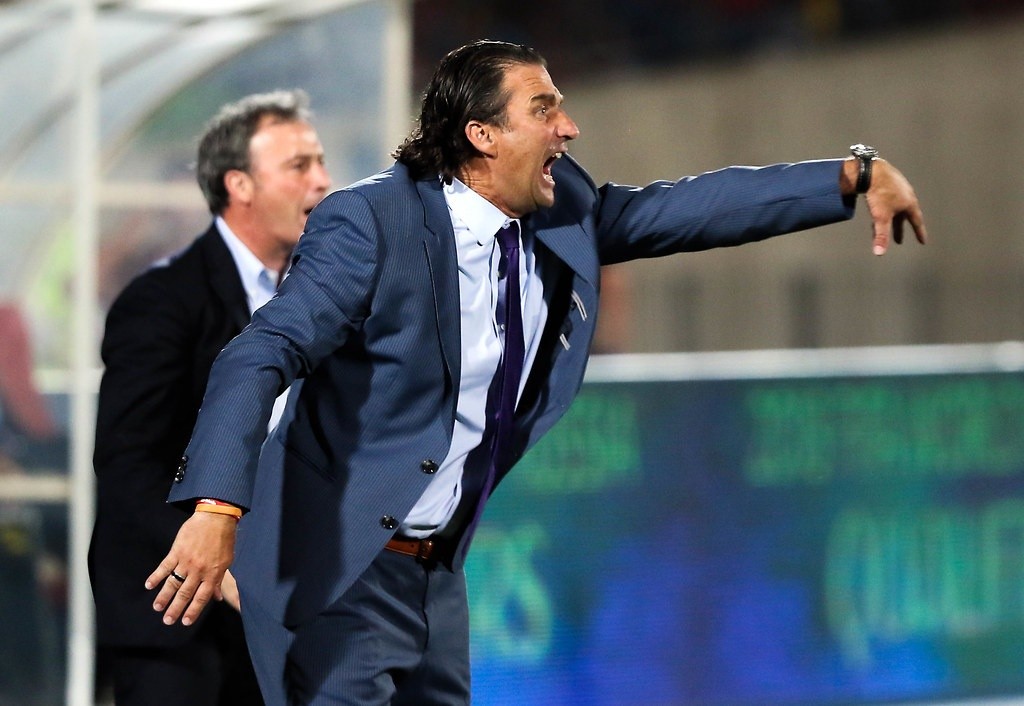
[{"xmin": 469, "ymin": 222, "xmax": 523, "ymax": 528}]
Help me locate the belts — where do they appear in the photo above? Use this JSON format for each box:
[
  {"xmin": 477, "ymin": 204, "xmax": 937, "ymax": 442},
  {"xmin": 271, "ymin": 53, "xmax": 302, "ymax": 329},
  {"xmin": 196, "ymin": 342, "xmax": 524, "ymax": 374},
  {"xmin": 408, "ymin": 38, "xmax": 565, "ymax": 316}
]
[{"xmin": 386, "ymin": 534, "xmax": 457, "ymax": 562}]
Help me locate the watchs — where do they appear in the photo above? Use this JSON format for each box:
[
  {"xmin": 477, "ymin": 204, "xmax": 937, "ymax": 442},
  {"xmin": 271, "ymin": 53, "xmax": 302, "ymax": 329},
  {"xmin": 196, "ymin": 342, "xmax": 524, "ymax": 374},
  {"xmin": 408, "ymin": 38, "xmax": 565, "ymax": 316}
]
[{"xmin": 849, "ymin": 144, "xmax": 879, "ymax": 194}]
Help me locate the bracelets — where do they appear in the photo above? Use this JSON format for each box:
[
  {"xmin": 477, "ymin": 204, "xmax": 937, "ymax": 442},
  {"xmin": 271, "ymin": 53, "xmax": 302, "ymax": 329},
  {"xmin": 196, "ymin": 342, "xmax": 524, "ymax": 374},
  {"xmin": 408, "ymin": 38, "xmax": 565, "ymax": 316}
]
[{"xmin": 193, "ymin": 497, "xmax": 243, "ymax": 520}]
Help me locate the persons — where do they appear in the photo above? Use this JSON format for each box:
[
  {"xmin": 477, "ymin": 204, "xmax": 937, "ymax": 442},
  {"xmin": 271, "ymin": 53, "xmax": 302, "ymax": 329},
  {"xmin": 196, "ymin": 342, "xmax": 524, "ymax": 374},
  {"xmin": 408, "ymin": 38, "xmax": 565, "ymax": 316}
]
[
  {"xmin": 86, "ymin": 89, "xmax": 330, "ymax": 706},
  {"xmin": 144, "ymin": 40, "xmax": 928, "ymax": 706}
]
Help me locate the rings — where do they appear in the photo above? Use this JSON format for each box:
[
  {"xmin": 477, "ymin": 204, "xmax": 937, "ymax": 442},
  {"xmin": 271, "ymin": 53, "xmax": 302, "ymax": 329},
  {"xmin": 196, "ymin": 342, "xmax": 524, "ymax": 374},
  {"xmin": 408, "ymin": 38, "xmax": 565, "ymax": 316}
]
[{"xmin": 171, "ymin": 571, "xmax": 185, "ymax": 583}]
